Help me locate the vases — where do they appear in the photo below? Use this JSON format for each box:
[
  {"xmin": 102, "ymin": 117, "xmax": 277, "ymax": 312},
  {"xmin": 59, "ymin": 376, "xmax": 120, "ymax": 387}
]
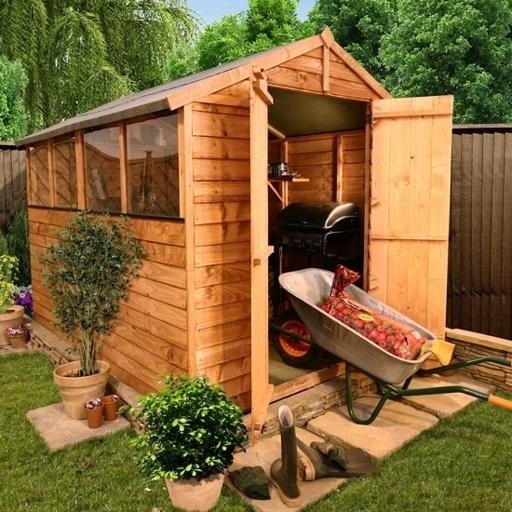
[
  {"xmin": 5, "ymin": 327, "xmax": 24, "ymax": 348},
  {"xmin": 85, "ymin": 394, "xmax": 119, "ymax": 430}
]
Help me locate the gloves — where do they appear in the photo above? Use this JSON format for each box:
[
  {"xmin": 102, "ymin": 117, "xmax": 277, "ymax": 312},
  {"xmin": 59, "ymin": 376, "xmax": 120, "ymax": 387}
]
[
  {"xmin": 228, "ymin": 462, "xmax": 271, "ymax": 501},
  {"xmin": 310, "ymin": 440, "xmax": 350, "ymax": 471}
]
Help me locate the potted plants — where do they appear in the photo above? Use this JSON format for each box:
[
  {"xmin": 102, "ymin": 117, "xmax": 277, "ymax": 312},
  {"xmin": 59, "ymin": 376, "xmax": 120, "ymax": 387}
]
[
  {"xmin": 0, "ymin": 253, "xmax": 24, "ymax": 348},
  {"xmin": 37, "ymin": 208, "xmax": 151, "ymax": 420},
  {"xmin": 116, "ymin": 376, "xmax": 246, "ymax": 511}
]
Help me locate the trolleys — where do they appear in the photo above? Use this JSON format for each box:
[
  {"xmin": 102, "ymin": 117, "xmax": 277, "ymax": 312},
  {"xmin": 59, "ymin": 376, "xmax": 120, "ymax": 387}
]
[{"xmin": 271, "ymin": 268, "xmax": 512, "ymax": 425}]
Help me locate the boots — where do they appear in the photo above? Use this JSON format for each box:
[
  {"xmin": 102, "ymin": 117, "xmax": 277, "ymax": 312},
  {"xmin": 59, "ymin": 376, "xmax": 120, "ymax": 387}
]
[
  {"xmin": 295, "ymin": 431, "xmax": 380, "ymax": 481},
  {"xmin": 268, "ymin": 405, "xmax": 302, "ymax": 507}
]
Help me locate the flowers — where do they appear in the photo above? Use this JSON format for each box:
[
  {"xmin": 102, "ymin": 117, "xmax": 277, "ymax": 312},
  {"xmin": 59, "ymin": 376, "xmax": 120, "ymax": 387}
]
[{"xmin": 15, "ymin": 291, "xmax": 32, "ymax": 313}]
